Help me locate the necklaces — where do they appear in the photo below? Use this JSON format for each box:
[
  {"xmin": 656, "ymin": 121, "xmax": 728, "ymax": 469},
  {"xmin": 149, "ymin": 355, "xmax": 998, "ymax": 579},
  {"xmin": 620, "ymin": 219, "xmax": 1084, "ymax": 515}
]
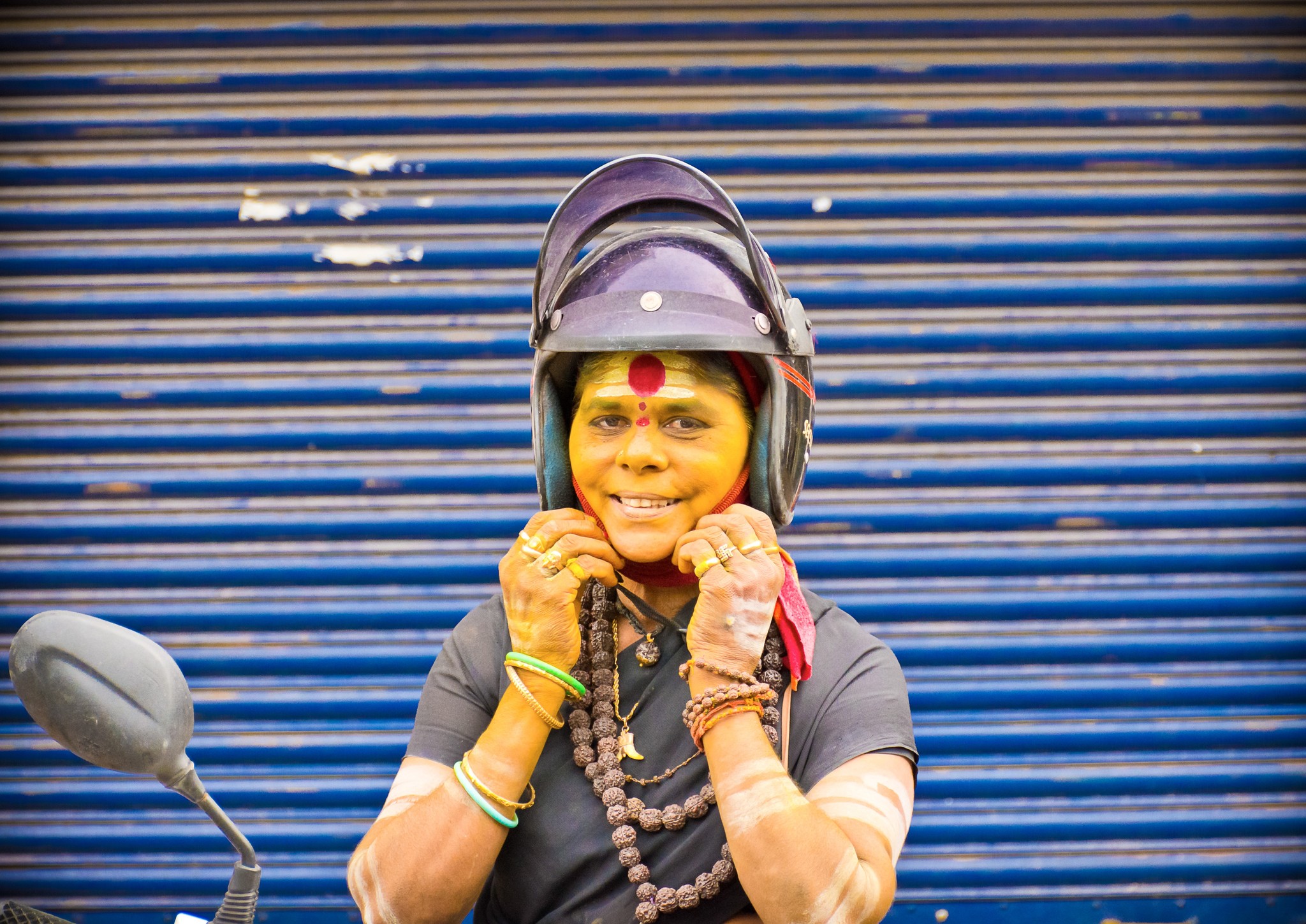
[{"xmin": 569, "ymin": 573, "xmax": 787, "ymax": 922}]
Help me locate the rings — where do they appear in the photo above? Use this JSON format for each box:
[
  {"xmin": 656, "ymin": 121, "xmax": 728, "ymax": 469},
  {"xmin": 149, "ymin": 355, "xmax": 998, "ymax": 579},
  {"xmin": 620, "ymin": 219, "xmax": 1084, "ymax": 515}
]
[
  {"xmin": 693, "ymin": 558, "xmax": 720, "ymax": 578},
  {"xmin": 541, "ymin": 547, "xmax": 563, "ymax": 570},
  {"xmin": 528, "ymin": 536, "xmax": 545, "ymax": 553},
  {"xmin": 716, "ymin": 543, "xmax": 739, "ymax": 563},
  {"xmin": 521, "ymin": 544, "xmax": 542, "ymax": 559},
  {"xmin": 565, "ymin": 557, "xmax": 586, "ymax": 583},
  {"xmin": 519, "ymin": 530, "xmax": 530, "ymax": 543},
  {"xmin": 739, "ymin": 541, "xmax": 764, "ymax": 555},
  {"xmin": 765, "ymin": 545, "xmax": 781, "ymax": 555}
]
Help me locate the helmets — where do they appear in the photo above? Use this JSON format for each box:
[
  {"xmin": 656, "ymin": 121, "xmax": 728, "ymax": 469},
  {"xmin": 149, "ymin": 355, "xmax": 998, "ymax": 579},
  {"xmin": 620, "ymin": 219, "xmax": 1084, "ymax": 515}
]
[{"xmin": 531, "ymin": 225, "xmax": 815, "ymax": 528}]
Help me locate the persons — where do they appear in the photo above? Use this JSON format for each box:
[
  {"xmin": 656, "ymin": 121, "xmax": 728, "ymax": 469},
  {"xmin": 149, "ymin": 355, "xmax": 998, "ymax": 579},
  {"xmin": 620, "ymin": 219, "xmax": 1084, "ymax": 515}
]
[{"xmin": 344, "ymin": 155, "xmax": 920, "ymax": 924}]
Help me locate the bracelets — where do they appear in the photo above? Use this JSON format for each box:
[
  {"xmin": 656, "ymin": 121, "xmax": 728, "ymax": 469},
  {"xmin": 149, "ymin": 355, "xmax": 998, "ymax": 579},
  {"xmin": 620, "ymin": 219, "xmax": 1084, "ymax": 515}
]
[
  {"xmin": 453, "ymin": 761, "xmax": 518, "ymax": 828},
  {"xmin": 462, "ymin": 749, "xmax": 535, "ymax": 810},
  {"xmin": 501, "ymin": 652, "xmax": 586, "ymax": 731}
]
